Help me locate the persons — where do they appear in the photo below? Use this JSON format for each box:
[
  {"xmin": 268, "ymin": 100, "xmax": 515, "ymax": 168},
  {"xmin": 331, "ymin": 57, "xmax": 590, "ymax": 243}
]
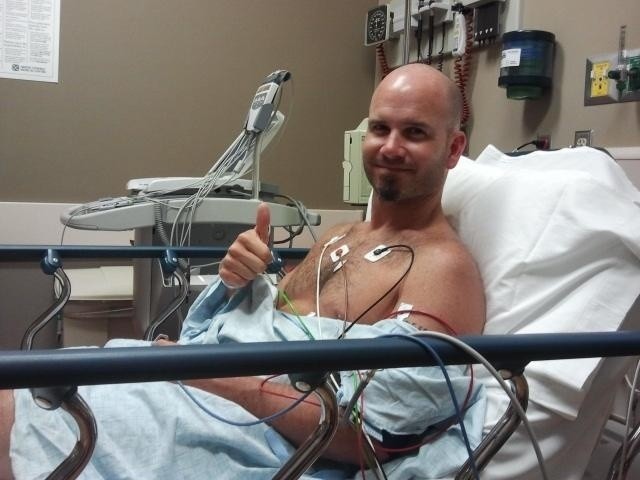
[{"xmin": 0, "ymin": 63, "xmax": 485, "ymax": 480}]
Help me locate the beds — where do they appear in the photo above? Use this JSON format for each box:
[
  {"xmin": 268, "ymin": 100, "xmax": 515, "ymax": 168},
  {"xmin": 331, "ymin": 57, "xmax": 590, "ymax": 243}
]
[{"xmin": 1, "ymin": 145, "xmax": 640, "ymax": 479}]
